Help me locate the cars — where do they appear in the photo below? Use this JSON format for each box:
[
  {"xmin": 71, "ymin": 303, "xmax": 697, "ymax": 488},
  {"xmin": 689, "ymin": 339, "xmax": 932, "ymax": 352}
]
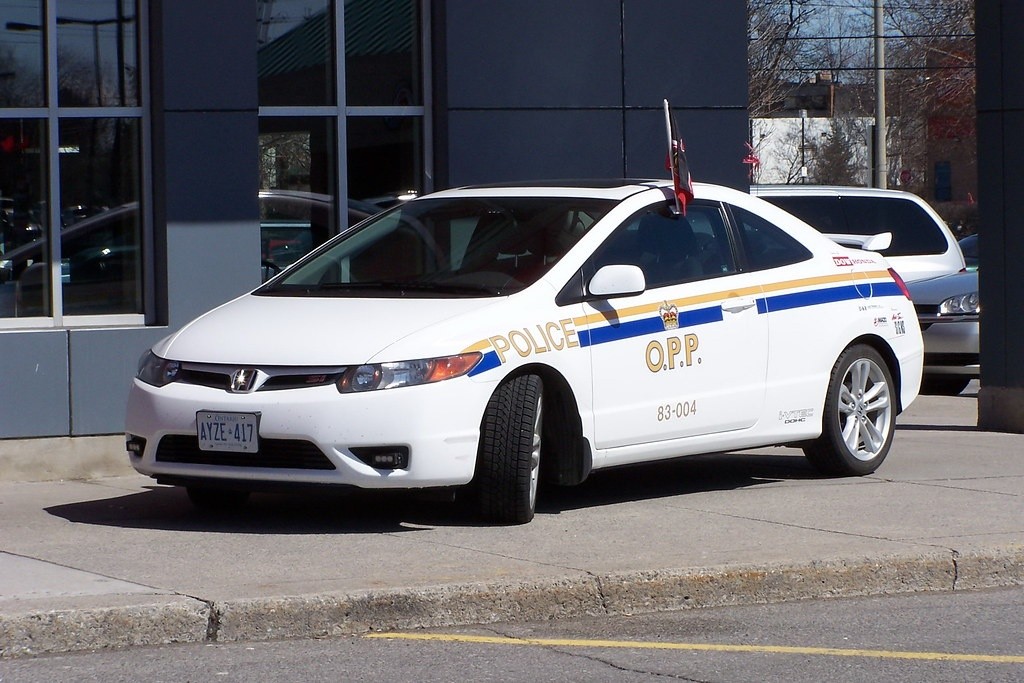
[
  {"xmin": 123, "ymin": 177, "xmax": 925, "ymax": 526},
  {"xmin": 902, "ymin": 231, "xmax": 980, "ymax": 396},
  {"xmin": 747, "ymin": 180, "xmax": 965, "ymax": 283},
  {"xmin": 1, "ymin": 183, "xmax": 450, "ymax": 318}
]
